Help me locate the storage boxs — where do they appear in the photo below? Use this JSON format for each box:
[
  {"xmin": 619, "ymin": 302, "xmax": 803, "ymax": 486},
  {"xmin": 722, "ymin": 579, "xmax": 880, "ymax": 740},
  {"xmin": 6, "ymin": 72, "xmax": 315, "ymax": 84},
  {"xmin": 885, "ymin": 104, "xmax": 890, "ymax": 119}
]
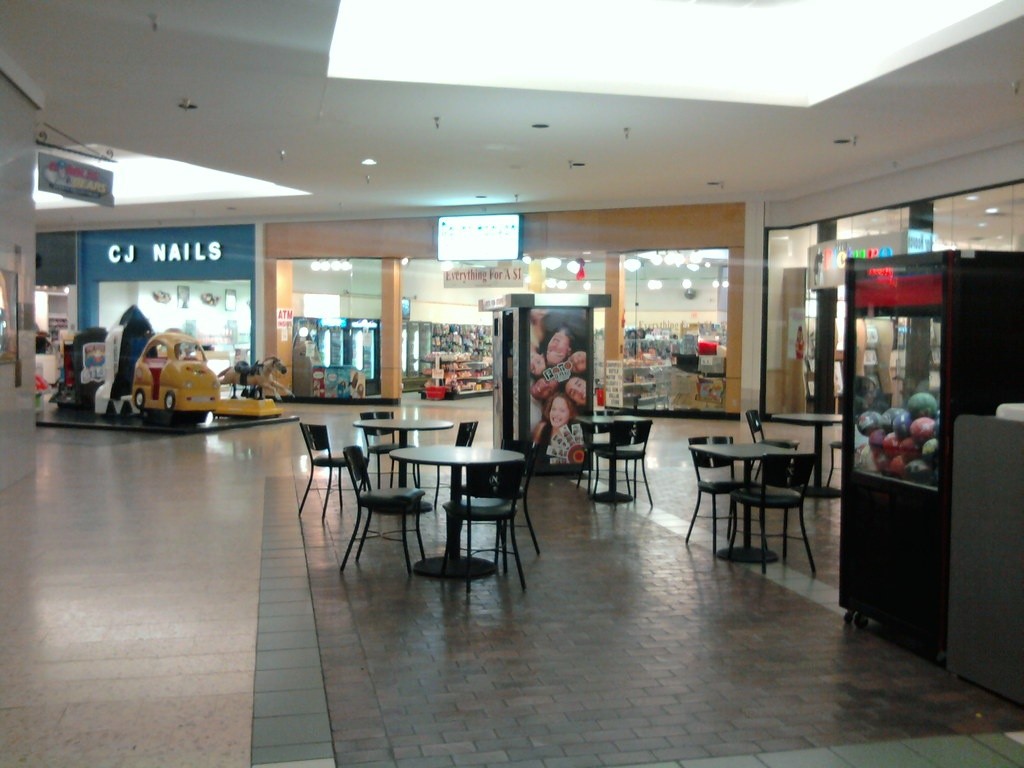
[
  {"xmin": 698, "ymin": 340, "xmax": 718, "ymax": 355},
  {"xmin": 673, "ymin": 353, "xmax": 700, "ymax": 374},
  {"xmin": 700, "ymin": 355, "xmax": 726, "ymax": 376},
  {"xmin": 425, "ymin": 386, "xmax": 445, "ymax": 400}
]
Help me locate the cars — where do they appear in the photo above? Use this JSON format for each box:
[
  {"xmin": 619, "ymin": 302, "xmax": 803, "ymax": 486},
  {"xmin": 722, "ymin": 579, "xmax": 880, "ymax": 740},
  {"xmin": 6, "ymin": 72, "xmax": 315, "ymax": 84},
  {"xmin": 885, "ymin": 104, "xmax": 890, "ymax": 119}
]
[{"xmin": 132, "ymin": 328, "xmax": 222, "ymax": 411}]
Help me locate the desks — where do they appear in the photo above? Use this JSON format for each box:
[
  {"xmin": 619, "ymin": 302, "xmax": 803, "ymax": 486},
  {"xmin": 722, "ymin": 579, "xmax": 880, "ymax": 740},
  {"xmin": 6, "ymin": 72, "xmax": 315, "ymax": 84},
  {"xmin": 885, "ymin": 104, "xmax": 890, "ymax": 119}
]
[
  {"xmin": 689, "ymin": 442, "xmax": 800, "ymax": 562},
  {"xmin": 771, "ymin": 412, "xmax": 843, "ymax": 498},
  {"xmin": 574, "ymin": 414, "xmax": 648, "ymax": 504},
  {"xmin": 388, "ymin": 445, "xmax": 528, "ymax": 579},
  {"xmin": 352, "ymin": 418, "xmax": 455, "ymax": 514}
]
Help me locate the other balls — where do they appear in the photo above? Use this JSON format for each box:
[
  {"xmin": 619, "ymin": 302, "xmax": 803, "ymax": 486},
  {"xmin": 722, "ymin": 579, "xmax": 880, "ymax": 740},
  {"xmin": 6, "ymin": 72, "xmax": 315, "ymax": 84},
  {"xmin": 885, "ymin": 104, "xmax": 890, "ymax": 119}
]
[{"xmin": 858, "ymin": 393, "xmax": 940, "ymax": 482}]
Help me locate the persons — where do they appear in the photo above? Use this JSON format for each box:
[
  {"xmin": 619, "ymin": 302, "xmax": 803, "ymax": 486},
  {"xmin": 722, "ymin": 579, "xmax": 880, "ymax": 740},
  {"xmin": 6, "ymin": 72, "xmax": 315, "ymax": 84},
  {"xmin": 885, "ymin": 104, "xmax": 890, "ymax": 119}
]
[
  {"xmin": 568, "ymin": 351, "xmax": 588, "ymax": 373},
  {"xmin": 564, "ymin": 375, "xmax": 586, "ymax": 407},
  {"xmin": 795, "ymin": 326, "xmax": 804, "ymax": 360},
  {"xmin": 626, "ymin": 328, "xmax": 636, "ymax": 355},
  {"xmin": 527, "ymin": 353, "xmax": 546, "ymax": 376},
  {"xmin": 530, "ymin": 307, "xmax": 588, "ymax": 364},
  {"xmin": 532, "ymin": 391, "xmax": 580, "ymax": 467},
  {"xmin": 637, "ymin": 328, "xmax": 648, "ymax": 353},
  {"xmin": 671, "ymin": 335, "xmax": 679, "ymax": 365},
  {"xmin": 529, "ymin": 376, "xmax": 559, "ymax": 435}
]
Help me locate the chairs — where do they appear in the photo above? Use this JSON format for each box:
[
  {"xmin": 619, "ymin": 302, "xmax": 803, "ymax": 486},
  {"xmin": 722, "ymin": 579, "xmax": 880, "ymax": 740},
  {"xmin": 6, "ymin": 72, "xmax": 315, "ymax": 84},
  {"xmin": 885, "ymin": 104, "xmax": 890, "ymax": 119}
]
[
  {"xmin": 340, "ymin": 445, "xmax": 429, "ymax": 576},
  {"xmin": 434, "ymin": 458, "xmax": 529, "ymax": 595},
  {"xmin": 745, "ymin": 410, "xmax": 800, "ymax": 481},
  {"xmin": 433, "ymin": 421, "xmax": 479, "ymax": 507},
  {"xmin": 684, "ymin": 435, "xmax": 769, "ymax": 554},
  {"xmin": 448, "ymin": 439, "xmax": 543, "ymax": 571},
  {"xmin": 826, "ymin": 441, "xmax": 842, "ymax": 487},
  {"xmin": 589, "ymin": 419, "xmax": 655, "ymax": 508},
  {"xmin": 725, "ymin": 452, "xmax": 817, "ymax": 577},
  {"xmin": 298, "ymin": 421, "xmax": 369, "ymax": 522},
  {"xmin": 359, "ymin": 411, "xmax": 421, "ymax": 488}
]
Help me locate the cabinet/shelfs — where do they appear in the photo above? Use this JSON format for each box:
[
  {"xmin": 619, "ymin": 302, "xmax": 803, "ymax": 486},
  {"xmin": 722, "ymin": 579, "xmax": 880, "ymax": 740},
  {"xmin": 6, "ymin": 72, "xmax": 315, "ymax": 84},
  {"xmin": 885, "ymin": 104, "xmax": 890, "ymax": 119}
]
[
  {"xmin": 439, "ymin": 354, "xmax": 493, "ymax": 401},
  {"xmin": 623, "ymin": 365, "xmax": 671, "ymax": 404},
  {"xmin": 418, "ymin": 355, "xmax": 436, "ymax": 398}
]
